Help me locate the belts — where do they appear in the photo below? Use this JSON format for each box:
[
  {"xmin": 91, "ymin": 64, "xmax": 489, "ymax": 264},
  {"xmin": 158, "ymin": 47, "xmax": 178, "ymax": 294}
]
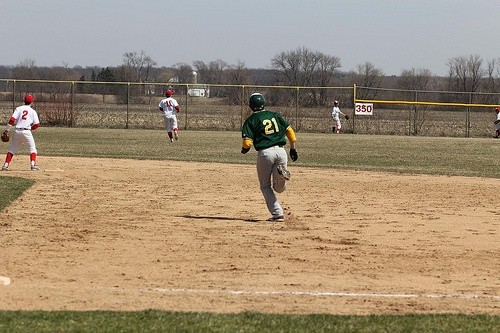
[
  {"xmin": 278, "ymin": 145, "xmax": 283, "ymax": 148},
  {"xmin": 20, "ymin": 128, "xmax": 30, "ymax": 130}
]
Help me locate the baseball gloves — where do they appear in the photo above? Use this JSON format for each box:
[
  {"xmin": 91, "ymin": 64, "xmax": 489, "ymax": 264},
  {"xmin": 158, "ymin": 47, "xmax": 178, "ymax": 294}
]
[
  {"xmin": 1, "ymin": 130, "xmax": 10, "ymax": 142},
  {"xmin": 345, "ymin": 115, "xmax": 349, "ymax": 120}
]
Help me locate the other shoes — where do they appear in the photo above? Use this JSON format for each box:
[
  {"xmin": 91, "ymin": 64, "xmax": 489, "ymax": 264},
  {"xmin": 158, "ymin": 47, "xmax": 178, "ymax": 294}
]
[
  {"xmin": 174, "ymin": 132, "xmax": 179, "ymax": 140},
  {"xmin": 30, "ymin": 165, "xmax": 40, "ymax": 170},
  {"xmin": 2, "ymin": 163, "xmax": 9, "ymax": 171},
  {"xmin": 171, "ymin": 139, "xmax": 174, "ymax": 142}
]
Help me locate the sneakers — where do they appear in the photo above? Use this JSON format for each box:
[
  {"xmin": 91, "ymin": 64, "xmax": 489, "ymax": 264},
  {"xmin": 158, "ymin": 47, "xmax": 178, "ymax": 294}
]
[
  {"xmin": 269, "ymin": 216, "xmax": 284, "ymax": 222},
  {"xmin": 277, "ymin": 165, "xmax": 291, "ymax": 181}
]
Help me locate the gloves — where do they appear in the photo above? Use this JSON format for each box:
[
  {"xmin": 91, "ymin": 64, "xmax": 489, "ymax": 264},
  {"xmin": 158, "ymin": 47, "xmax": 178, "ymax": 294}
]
[
  {"xmin": 240, "ymin": 147, "xmax": 248, "ymax": 155},
  {"xmin": 290, "ymin": 148, "xmax": 299, "ymax": 162}
]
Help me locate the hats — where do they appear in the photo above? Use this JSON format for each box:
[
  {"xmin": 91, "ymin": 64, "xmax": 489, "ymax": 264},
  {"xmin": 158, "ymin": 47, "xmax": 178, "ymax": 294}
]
[
  {"xmin": 334, "ymin": 101, "xmax": 340, "ymax": 105},
  {"xmin": 166, "ymin": 90, "xmax": 173, "ymax": 95},
  {"xmin": 24, "ymin": 95, "xmax": 34, "ymax": 102},
  {"xmin": 494, "ymin": 108, "xmax": 500, "ymax": 112}
]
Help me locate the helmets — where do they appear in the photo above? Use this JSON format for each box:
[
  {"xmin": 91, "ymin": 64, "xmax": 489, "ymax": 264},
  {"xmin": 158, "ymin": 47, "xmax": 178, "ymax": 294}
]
[{"xmin": 249, "ymin": 93, "xmax": 265, "ymax": 110}]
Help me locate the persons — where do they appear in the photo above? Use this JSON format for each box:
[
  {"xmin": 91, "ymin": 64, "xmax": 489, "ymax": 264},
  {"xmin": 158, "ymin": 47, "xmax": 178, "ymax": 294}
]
[
  {"xmin": 2, "ymin": 95, "xmax": 41, "ymax": 170},
  {"xmin": 158, "ymin": 89, "xmax": 180, "ymax": 142},
  {"xmin": 332, "ymin": 100, "xmax": 348, "ymax": 134},
  {"xmin": 493, "ymin": 107, "xmax": 500, "ymax": 138},
  {"xmin": 241, "ymin": 93, "xmax": 298, "ymax": 221}
]
